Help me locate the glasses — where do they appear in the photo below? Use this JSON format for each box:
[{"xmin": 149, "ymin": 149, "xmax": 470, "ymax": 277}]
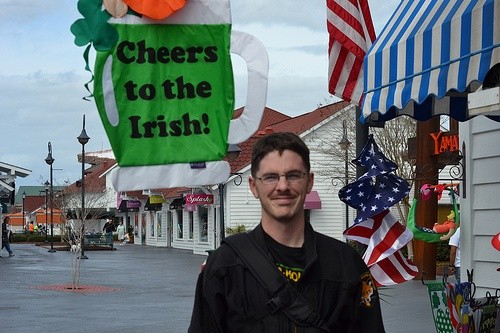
[{"xmin": 252, "ymin": 171, "xmax": 308, "ymax": 184}]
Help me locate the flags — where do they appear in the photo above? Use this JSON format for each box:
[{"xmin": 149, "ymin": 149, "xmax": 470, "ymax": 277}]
[{"xmin": 327, "ymin": 0, "xmax": 377, "ymax": 109}]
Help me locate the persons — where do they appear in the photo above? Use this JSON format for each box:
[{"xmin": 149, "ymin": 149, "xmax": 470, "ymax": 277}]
[
  {"xmin": 103, "ymin": 218, "xmax": 116, "ymax": 244},
  {"xmin": 185, "ymin": 132, "xmax": 385, "ymax": 333},
  {"xmin": 417, "ymin": 214, "xmax": 456, "ymax": 240},
  {"xmin": 26, "ymin": 221, "xmax": 46, "ymax": 234},
  {"xmin": 116, "ymin": 221, "xmax": 125, "ymax": 243},
  {"xmin": 2, "ymin": 216, "xmax": 15, "ymax": 257},
  {"xmin": 448, "ymin": 226, "xmax": 460, "ymax": 284}
]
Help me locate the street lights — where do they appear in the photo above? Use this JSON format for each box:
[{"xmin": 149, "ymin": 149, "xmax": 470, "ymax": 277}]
[
  {"xmin": 77, "ymin": 114, "xmax": 90, "ymax": 259},
  {"xmin": 44, "ymin": 142, "xmax": 56, "ymax": 252},
  {"xmin": 22, "ymin": 191, "xmax": 26, "ymax": 229},
  {"xmin": 338, "ymin": 120, "xmax": 352, "ymax": 244},
  {"xmin": 219, "ymin": 145, "xmax": 244, "ymax": 242}
]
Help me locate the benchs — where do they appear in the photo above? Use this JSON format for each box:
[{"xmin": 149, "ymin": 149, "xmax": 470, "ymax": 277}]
[{"xmin": 84, "ymin": 232, "xmax": 114, "ymax": 250}]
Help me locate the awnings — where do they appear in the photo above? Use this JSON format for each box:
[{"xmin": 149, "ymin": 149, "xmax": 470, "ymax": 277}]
[{"xmin": 360, "ymin": 0, "xmax": 500, "ymax": 126}]
